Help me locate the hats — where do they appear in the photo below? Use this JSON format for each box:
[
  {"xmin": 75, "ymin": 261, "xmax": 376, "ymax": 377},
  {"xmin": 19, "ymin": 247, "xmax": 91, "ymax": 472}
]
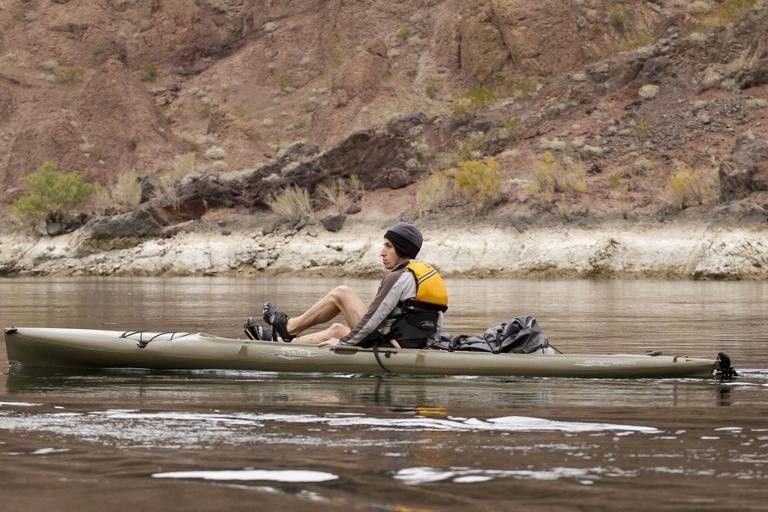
[{"xmin": 384, "ymin": 223, "xmax": 422, "ymax": 259}]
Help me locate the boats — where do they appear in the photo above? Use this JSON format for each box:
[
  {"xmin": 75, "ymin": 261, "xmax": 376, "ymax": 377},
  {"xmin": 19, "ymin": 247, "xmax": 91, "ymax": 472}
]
[{"xmin": 4, "ymin": 326, "xmax": 730, "ymax": 381}]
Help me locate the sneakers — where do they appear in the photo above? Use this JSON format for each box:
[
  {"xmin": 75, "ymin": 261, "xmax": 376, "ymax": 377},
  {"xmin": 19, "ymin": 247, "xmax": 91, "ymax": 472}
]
[
  {"xmin": 263, "ymin": 301, "xmax": 296, "ymax": 342},
  {"xmin": 247, "ymin": 317, "xmax": 272, "ymax": 341}
]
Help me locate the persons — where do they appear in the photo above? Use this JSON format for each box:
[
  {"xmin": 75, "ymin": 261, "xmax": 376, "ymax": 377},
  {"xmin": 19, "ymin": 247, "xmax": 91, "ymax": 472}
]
[
  {"xmin": 243, "ymin": 224, "xmax": 447, "ymax": 350},
  {"xmin": 258, "ymin": 382, "xmax": 450, "ymax": 417}
]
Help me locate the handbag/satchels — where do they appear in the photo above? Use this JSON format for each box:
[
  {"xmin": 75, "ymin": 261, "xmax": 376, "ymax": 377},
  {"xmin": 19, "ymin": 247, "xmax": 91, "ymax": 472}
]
[{"xmin": 432, "ymin": 316, "xmax": 548, "ymax": 354}]
